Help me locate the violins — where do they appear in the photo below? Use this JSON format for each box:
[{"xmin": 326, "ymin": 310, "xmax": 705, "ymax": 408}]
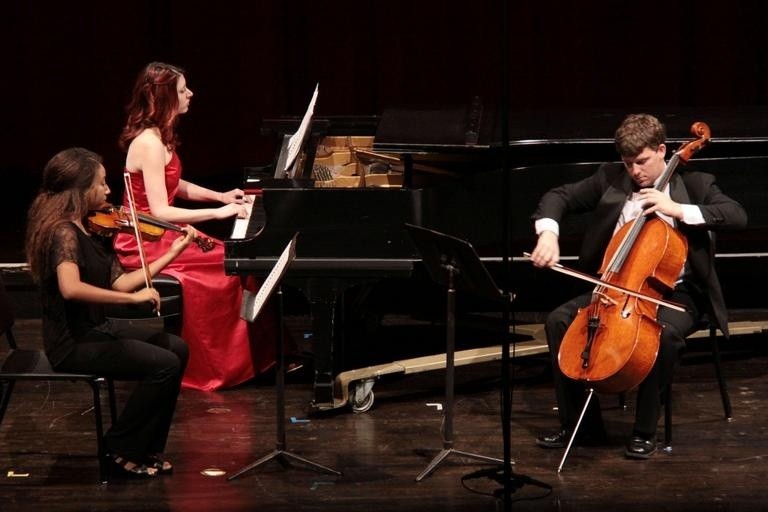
[{"xmin": 87, "ymin": 204, "xmax": 216, "ymax": 252}]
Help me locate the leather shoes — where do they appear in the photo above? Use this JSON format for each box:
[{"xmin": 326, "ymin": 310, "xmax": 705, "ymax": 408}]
[
  {"xmin": 625, "ymin": 432, "xmax": 658, "ymax": 458},
  {"xmin": 535, "ymin": 424, "xmax": 570, "ymax": 448}
]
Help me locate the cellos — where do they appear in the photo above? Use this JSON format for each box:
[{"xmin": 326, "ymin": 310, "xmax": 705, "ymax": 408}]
[{"xmin": 558, "ymin": 122, "xmax": 710, "ymax": 392}]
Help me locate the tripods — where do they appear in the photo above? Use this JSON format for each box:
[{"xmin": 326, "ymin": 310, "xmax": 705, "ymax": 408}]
[
  {"xmin": 387, "ymin": 268, "xmax": 515, "ymax": 480},
  {"xmin": 227, "ymin": 270, "xmax": 342, "ymax": 479}
]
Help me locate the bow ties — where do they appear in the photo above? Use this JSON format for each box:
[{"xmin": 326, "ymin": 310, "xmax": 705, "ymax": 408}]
[{"xmin": 633, "ymin": 184, "xmax": 653, "ymax": 192}]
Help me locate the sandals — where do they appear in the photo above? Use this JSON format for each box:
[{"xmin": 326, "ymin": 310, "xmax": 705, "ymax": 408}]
[{"xmin": 102, "ymin": 451, "xmax": 174, "ymax": 478}]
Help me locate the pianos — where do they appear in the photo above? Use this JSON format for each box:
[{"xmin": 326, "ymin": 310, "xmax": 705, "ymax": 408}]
[{"xmin": 224, "ymin": 179, "xmax": 421, "ymax": 419}]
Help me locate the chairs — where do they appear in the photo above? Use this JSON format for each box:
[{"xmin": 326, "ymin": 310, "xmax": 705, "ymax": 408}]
[
  {"xmin": 0, "ymin": 262, "xmax": 117, "ymax": 485},
  {"xmin": 587, "ymin": 223, "xmax": 734, "ymax": 453},
  {"xmin": 116, "ymin": 265, "xmax": 183, "ymax": 334}
]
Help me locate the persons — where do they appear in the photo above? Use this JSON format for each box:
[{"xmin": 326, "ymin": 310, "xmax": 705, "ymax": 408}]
[
  {"xmin": 531, "ymin": 114, "xmax": 748, "ymax": 458},
  {"xmin": 25, "ymin": 149, "xmax": 189, "ymax": 480},
  {"xmin": 113, "ymin": 61, "xmax": 296, "ymax": 394}
]
